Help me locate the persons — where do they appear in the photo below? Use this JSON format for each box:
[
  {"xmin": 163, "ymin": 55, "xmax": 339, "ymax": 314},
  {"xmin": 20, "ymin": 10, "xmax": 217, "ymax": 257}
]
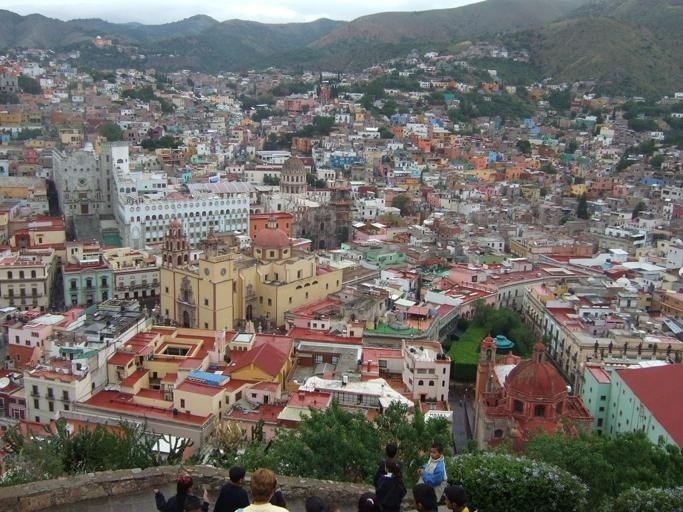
[
  {"xmin": 411, "ymin": 484, "xmax": 438, "ymax": 512},
  {"xmin": 443, "ymin": 486, "xmax": 475, "ymax": 512},
  {"xmin": 269, "ymin": 477, "xmax": 286, "ymax": 507},
  {"xmin": 326, "ymin": 504, "xmax": 340, "ymax": 512},
  {"xmin": 234, "ymin": 469, "xmax": 289, "ymax": 512},
  {"xmin": 355, "ymin": 492, "xmax": 380, "ymax": 512},
  {"xmin": 152, "ymin": 476, "xmax": 210, "ymax": 512},
  {"xmin": 183, "ymin": 494, "xmax": 202, "ymax": 512},
  {"xmin": 376, "ymin": 461, "xmax": 407, "ymax": 512},
  {"xmin": 212, "ymin": 466, "xmax": 249, "ymax": 512},
  {"xmin": 373, "ymin": 444, "xmax": 402, "ymax": 487},
  {"xmin": 304, "ymin": 496, "xmax": 324, "ymax": 512},
  {"xmin": 414, "ymin": 443, "xmax": 447, "ymax": 503}
]
